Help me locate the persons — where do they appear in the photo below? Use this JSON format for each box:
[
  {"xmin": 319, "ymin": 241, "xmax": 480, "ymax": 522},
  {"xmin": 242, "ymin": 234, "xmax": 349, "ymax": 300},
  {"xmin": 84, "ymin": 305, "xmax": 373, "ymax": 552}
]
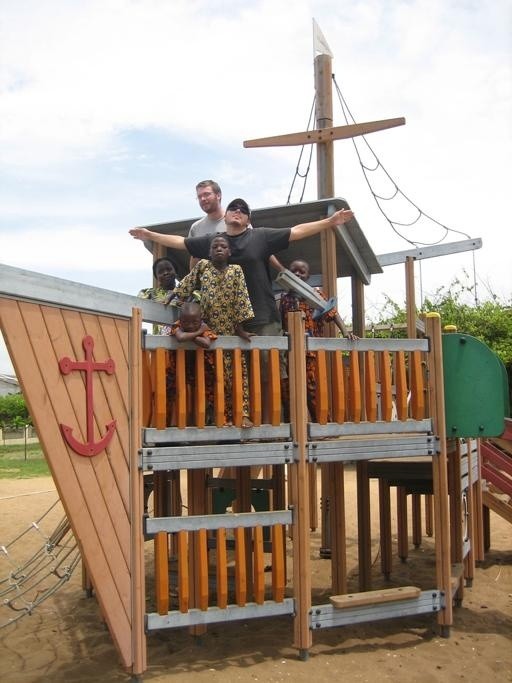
[
  {"xmin": 186, "ymin": 177, "xmax": 254, "ymax": 274},
  {"xmin": 280, "ymin": 258, "xmax": 360, "ymax": 423},
  {"xmin": 136, "ymin": 237, "xmax": 256, "ymax": 427},
  {"xmin": 128, "ymin": 197, "xmax": 355, "ymax": 424}
]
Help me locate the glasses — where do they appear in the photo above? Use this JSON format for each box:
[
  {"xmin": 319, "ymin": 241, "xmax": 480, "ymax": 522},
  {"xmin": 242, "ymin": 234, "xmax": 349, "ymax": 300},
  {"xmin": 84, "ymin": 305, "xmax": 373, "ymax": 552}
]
[{"xmin": 228, "ymin": 203, "xmax": 249, "ymax": 214}]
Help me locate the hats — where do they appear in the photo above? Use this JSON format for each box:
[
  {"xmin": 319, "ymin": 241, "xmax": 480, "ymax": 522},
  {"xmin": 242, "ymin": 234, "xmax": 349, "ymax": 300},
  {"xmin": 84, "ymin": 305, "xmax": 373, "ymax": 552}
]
[{"xmin": 226, "ymin": 198, "xmax": 248, "ymax": 212}]
